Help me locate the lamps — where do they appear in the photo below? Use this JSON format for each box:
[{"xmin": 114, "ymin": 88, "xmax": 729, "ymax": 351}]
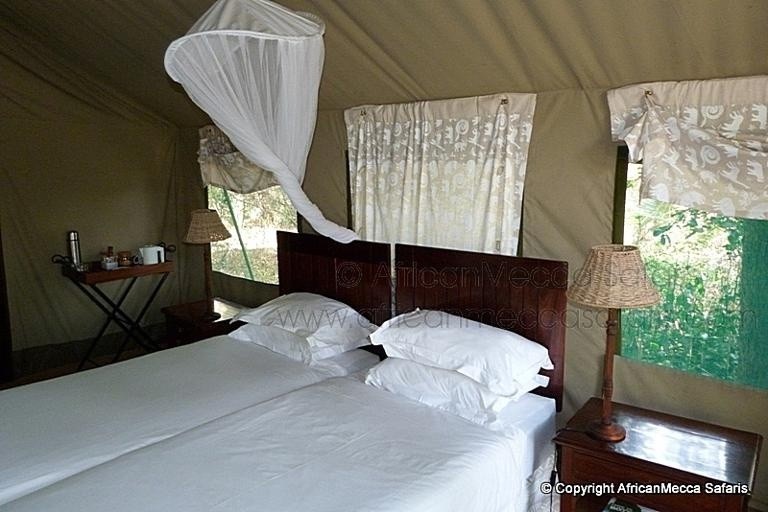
[
  {"xmin": 181, "ymin": 209, "xmax": 233, "ymax": 321},
  {"xmin": 564, "ymin": 243, "xmax": 660, "ymax": 442}
]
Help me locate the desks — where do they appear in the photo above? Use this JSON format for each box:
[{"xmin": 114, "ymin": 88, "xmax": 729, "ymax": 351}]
[{"xmin": 63, "ymin": 260, "xmax": 184, "ymax": 371}]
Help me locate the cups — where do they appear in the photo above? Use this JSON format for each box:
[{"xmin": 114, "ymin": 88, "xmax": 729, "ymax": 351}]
[{"xmin": 68, "ymin": 231, "xmax": 81, "ymax": 264}]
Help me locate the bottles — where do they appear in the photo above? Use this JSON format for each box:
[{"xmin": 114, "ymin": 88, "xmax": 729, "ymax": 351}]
[{"xmin": 101, "ymin": 255, "xmax": 118, "ymax": 270}]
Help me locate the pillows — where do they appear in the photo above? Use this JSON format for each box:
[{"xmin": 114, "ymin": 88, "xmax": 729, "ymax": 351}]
[
  {"xmin": 368, "ymin": 306, "xmax": 556, "ymax": 396},
  {"xmin": 230, "ymin": 321, "xmax": 376, "ymax": 364},
  {"xmin": 363, "ymin": 355, "xmax": 552, "ymax": 428},
  {"xmin": 240, "ymin": 293, "xmax": 380, "ymax": 348}
]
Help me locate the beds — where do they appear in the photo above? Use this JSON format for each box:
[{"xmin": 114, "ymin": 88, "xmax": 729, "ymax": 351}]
[{"xmin": 0, "ymin": 226, "xmax": 579, "ymax": 511}]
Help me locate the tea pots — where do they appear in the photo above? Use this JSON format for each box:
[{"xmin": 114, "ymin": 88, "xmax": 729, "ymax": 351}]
[{"xmin": 139, "ymin": 244, "xmax": 165, "ymax": 265}]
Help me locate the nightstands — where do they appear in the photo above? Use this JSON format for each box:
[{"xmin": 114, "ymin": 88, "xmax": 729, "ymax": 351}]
[
  {"xmin": 162, "ymin": 296, "xmax": 255, "ymax": 344},
  {"xmin": 549, "ymin": 396, "xmax": 764, "ymax": 512}
]
